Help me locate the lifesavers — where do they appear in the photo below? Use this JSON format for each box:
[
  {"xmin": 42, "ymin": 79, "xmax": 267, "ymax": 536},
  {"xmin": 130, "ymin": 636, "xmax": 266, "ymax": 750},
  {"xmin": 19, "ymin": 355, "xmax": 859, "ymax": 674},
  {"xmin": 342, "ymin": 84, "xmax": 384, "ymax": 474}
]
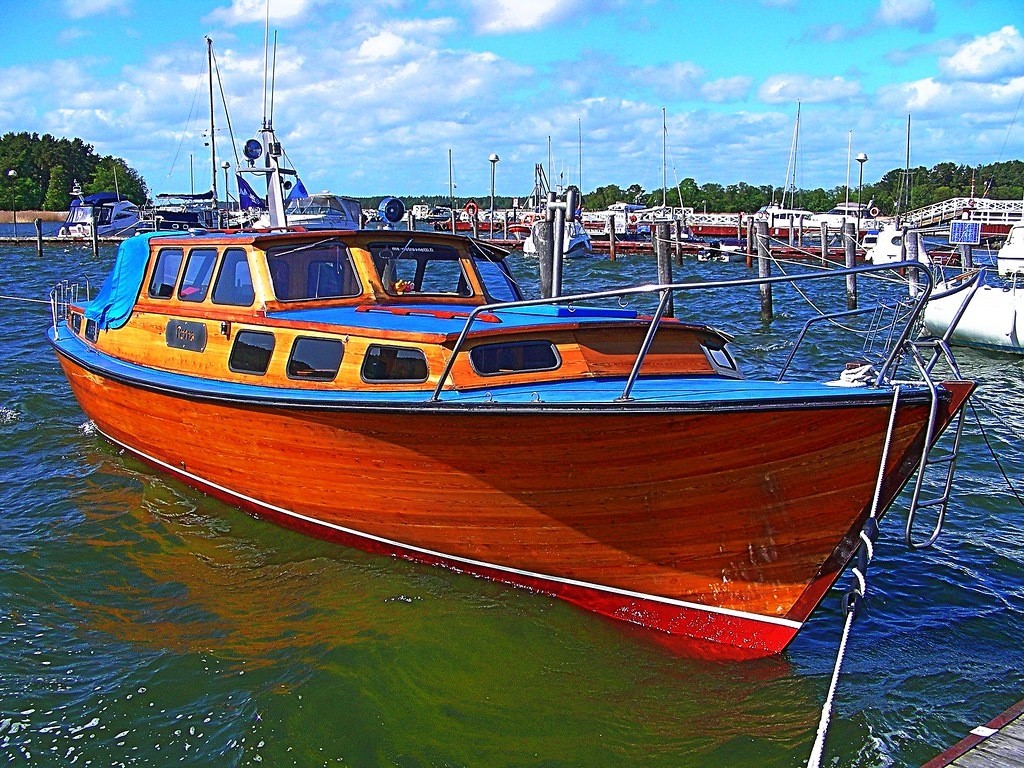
[
  {"xmin": 465, "ymin": 203, "xmax": 479, "ymax": 216},
  {"xmin": 870, "ymin": 207, "xmax": 880, "ymax": 217}
]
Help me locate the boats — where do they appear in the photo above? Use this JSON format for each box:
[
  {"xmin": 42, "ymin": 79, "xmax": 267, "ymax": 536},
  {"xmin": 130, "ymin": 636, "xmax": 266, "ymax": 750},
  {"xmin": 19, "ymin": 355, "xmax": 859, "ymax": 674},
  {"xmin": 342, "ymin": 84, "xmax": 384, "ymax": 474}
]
[
  {"xmin": 505, "ymin": 163, "xmax": 550, "ymax": 240},
  {"xmin": 58, "ymin": 166, "xmax": 141, "ymax": 236},
  {"xmin": 138, "ymin": 155, "xmax": 221, "ymax": 231},
  {"xmin": 47, "ymin": 0, "xmax": 985, "ymax": 657},
  {"xmin": 918, "ymin": 220, "xmax": 1024, "ymax": 355},
  {"xmin": 809, "ymin": 201, "xmax": 881, "ymax": 230}
]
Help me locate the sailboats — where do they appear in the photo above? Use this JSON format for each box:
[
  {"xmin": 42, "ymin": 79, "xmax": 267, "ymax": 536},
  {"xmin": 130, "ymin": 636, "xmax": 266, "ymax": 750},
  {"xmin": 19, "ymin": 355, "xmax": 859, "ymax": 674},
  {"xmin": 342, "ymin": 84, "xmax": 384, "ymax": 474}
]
[
  {"xmin": 754, "ymin": 99, "xmax": 816, "ymax": 228},
  {"xmin": 424, "ymin": 149, "xmax": 459, "ymax": 222},
  {"xmin": 606, "ymin": 107, "xmax": 693, "ymax": 235}
]
